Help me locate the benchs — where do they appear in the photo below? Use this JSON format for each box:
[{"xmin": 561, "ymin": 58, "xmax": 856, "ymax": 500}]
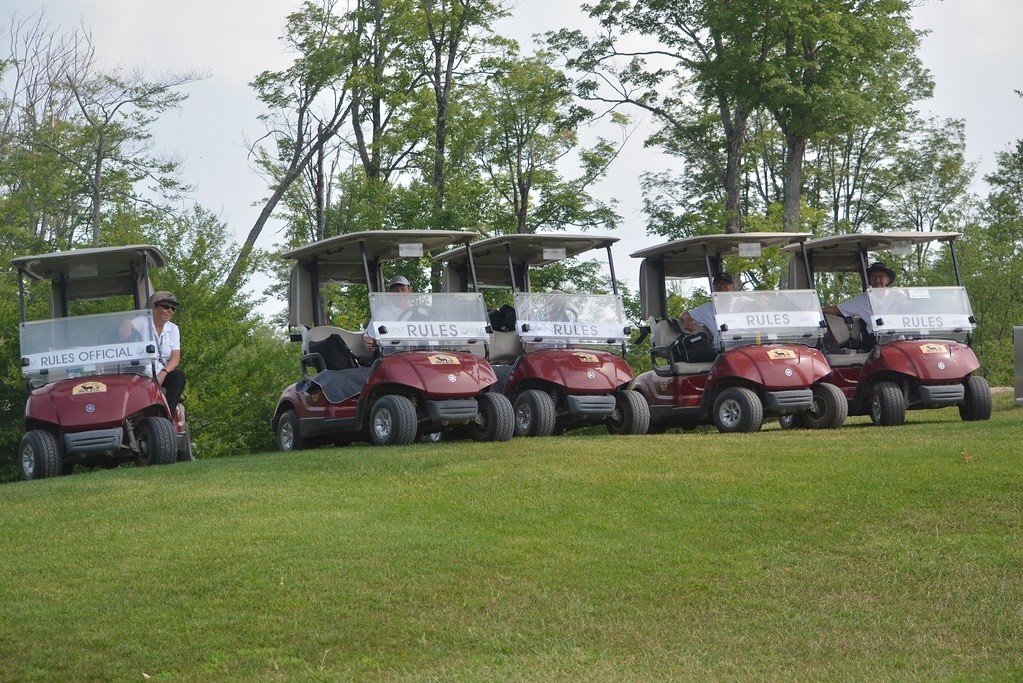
[
  {"xmin": 652, "ymin": 317, "xmax": 715, "ymax": 359},
  {"xmin": 820, "ymin": 312, "xmax": 876, "ymax": 367},
  {"xmin": 461, "ymin": 327, "xmax": 543, "ymax": 362},
  {"xmin": 300, "ymin": 325, "xmax": 374, "ymax": 365}
]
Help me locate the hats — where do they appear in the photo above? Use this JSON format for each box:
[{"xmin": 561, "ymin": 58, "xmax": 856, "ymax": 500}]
[
  {"xmin": 713, "ymin": 272, "xmax": 733, "ymax": 284},
  {"xmin": 866, "ymin": 262, "xmax": 895, "ymax": 287},
  {"xmin": 389, "ymin": 276, "xmax": 410, "ymax": 288},
  {"xmin": 153, "ymin": 291, "xmax": 180, "ymax": 307}
]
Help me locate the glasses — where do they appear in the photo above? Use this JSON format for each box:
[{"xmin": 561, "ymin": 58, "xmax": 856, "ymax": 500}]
[{"xmin": 155, "ymin": 303, "xmax": 175, "ymax": 312}]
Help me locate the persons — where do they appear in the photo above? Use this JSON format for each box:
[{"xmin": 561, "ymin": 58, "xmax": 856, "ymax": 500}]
[
  {"xmin": 361, "ymin": 276, "xmax": 435, "ymax": 357},
  {"xmin": 823, "ymin": 262, "xmax": 906, "ymax": 349},
  {"xmin": 119, "ymin": 291, "xmax": 189, "ymax": 437},
  {"xmin": 680, "ymin": 272, "xmax": 768, "ymax": 350}
]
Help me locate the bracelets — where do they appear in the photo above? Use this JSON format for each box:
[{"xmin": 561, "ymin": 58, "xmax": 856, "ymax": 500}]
[{"xmin": 161, "ymin": 369, "xmax": 169, "ymax": 374}]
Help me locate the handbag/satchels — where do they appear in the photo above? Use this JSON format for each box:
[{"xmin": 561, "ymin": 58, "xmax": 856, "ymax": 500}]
[
  {"xmin": 488, "ymin": 304, "xmax": 516, "ymax": 332},
  {"xmin": 672, "ymin": 332, "xmax": 717, "ymax": 364}
]
